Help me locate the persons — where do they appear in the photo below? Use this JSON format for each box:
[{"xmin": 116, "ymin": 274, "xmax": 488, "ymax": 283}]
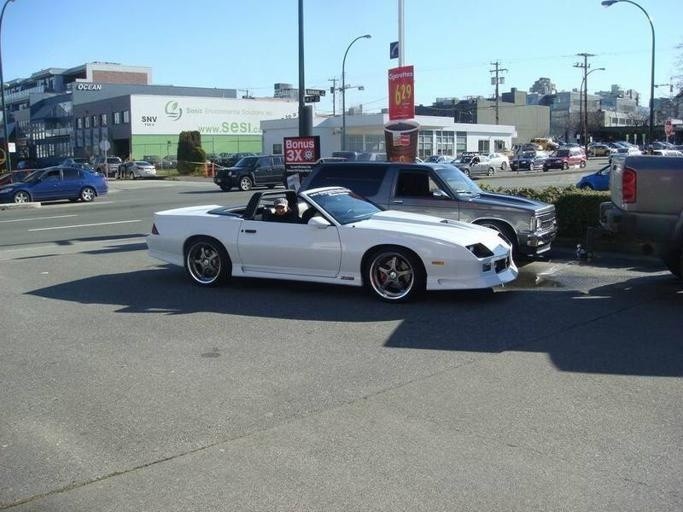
[{"xmin": 268, "ymin": 198, "xmax": 301, "ymax": 226}]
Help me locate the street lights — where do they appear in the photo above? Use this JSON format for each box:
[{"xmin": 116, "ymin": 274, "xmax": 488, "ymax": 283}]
[
  {"xmin": 600, "ymin": 1, "xmax": 655, "ymax": 156},
  {"xmin": 579, "ymin": 68, "xmax": 605, "ymax": 147},
  {"xmin": 0, "ymin": 0, "xmax": 16, "ymax": 172},
  {"xmin": 0, "ymin": 82, "xmax": 11, "ymax": 91},
  {"xmin": 342, "ymin": 34, "xmax": 372, "ymax": 152}
]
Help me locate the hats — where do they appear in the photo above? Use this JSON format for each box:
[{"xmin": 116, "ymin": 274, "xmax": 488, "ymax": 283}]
[{"xmin": 274, "ymin": 198, "xmax": 288, "ymax": 208}]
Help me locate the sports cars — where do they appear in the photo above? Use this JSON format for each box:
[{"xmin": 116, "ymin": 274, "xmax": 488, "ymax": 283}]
[{"xmin": 145, "ymin": 186, "xmax": 519, "ymax": 304}]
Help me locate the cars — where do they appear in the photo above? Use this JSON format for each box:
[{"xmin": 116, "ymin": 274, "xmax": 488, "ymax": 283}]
[
  {"xmin": 415, "ymin": 157, "xmax": 423, "ymax": 163},
  {"xmin": 205, "ymin": 151, "xmax": 266, "ymax": 169},
  {"xmin": 118, "ymin": 161, "xmax": 157, "ymax": 180},
  {"xmin": 511, "ymin": 137, "xmax": 683, "ymax": 156},
  {"xmin": 509, "ymin": 150, "xmax": 549, "ymax": 171},
  {"xmin": 450, "ymin": 156, "xmax": 497, "ymax": 177},
  {"xmin": 0, "ymin": 166, "xmax": 107, "ymax": 204},
  {"xmin": 162, "ymin": 155, "xmax": 178, "ymax": 169},
  {"xmin": 57, "ymin": 157, "xmax": 96, "ymax": 170},
  {"xmin": 17, "ymin": 160, "xmax": 41, "ymax": 170},
  {"xmin": 426, "ymin": 156, "xmax": 454, "ymax": 164},
  {"xmin": 143, "ymin": 155, "xmax": 172, "ymax": 170},
  {"xmin": 480, "ymin": 152, "xmax": 511, "ymax": 171},
  {"xmin": 332, "ymin": 151, "xmax": 387, "ymax": 161}
]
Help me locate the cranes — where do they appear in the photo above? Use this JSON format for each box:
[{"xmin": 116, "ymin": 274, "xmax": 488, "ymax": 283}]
[{"xmin": 653, "ymin": 76, "xmax": 680, "ymax": 119}]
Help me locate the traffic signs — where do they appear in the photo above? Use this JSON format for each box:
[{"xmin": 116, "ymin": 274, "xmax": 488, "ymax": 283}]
[
  {"xmin": 303, "ymin": 96, "xmax": 321, "ymax": 102},
  {"xmin": 306, "ymin": 89, "xmax": 326, "ymax": 96}
]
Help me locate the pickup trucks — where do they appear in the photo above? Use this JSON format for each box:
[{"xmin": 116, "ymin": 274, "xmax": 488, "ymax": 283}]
[{"xmin": 599, "ymin": 154, "xmax": 683, "ymax": 281}]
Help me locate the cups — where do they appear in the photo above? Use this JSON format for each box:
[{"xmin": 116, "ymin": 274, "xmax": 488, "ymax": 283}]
[{"xmin": 383, "ymin": 118, "xmax": 420, "ymax": 164}]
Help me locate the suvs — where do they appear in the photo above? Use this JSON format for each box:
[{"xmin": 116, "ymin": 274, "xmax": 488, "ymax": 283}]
[
  {"xmin": 542, "ymin": 147, "xmax": 587, "ymax": 171},
  {"xmin": 213, "ymin": 153, "xmax": 287, "ymax": 191},
  {"xmin": 96, "ymin": 156, "xmax": 124, "ymax": 176},
  {"xmin": 296, "ymin": 158, "xmax": 558, "ymax": 257}
]
